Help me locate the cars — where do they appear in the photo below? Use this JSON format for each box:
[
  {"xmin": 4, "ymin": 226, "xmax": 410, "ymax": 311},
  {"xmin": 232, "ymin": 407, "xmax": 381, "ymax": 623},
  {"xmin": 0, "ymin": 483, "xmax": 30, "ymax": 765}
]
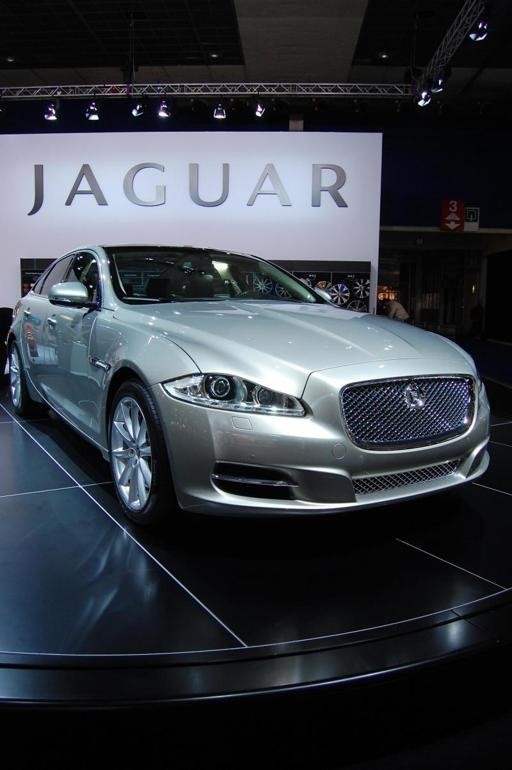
[{"xmin": 3, "ymin": 242, "xmax": 495, "ymax": 531}]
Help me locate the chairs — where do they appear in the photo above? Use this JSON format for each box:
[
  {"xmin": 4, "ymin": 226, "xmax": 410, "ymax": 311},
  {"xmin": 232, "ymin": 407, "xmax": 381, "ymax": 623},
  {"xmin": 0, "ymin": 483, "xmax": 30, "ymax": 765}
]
[{"xmin": 146, "ymin": 271, "xmax": 214, "ymax": 299}]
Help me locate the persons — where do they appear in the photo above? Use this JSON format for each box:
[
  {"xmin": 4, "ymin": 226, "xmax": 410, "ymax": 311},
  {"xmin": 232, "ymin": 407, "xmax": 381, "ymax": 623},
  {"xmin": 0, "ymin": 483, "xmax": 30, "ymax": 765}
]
[{"xmin": 381, "ymin": 298, "xmax": 409, "ymax": 324}]
